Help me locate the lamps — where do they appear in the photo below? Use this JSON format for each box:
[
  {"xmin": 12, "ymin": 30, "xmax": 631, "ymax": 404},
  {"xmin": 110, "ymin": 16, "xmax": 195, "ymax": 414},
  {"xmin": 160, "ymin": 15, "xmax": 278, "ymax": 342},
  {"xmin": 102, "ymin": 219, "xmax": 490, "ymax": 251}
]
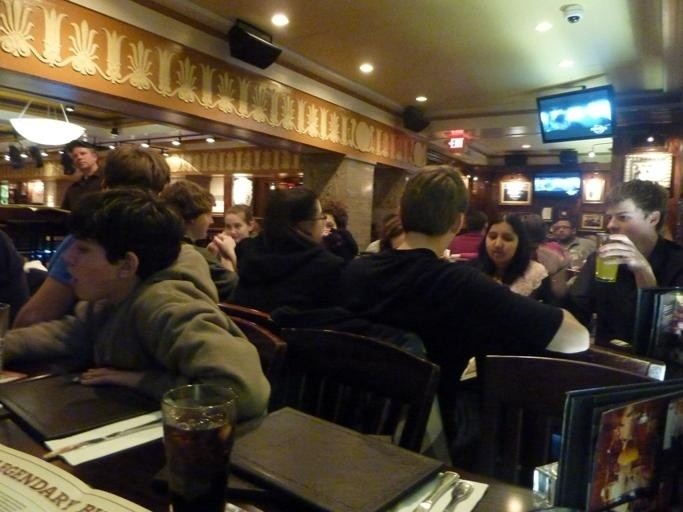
[
  {"xmin": 8, "ymin": 95, "xmax": 89, "ymax": 147},
  {"xmin": 0, "ymin": 126, "xmax": 220, "ymax": 175}
]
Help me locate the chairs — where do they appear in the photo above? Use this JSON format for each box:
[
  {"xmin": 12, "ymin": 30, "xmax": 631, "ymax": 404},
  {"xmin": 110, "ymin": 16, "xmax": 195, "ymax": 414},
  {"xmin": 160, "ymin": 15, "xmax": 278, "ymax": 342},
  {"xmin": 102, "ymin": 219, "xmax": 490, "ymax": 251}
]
[
  {"xmin": 218, "ymin": 302, "xmax": 276, "ymax": 334},
  {"xmin": 225, "ymin": 316, "xmax": 296, "ymax": 421},
  {"xmin": 467, "ymin": 349, "xmax": 668, "ymax": 496},
  {"xmin": 279, "ymin": 318, "xmax": 445, "ymax": 458}
]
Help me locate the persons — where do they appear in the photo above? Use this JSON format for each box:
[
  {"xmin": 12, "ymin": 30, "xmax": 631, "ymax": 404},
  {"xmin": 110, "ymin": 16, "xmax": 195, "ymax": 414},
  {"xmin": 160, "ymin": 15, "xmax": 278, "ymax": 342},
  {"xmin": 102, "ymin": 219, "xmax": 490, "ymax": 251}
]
[
  {"xmin": 0, "ymin": 186, "xmax": 272, "ymax": 424},
  {"xmin": 1, "ymin": 141, "xmax": 682, "ymax": 360},
  {"xmin": 332, "ymin": 162, "xmax": 592, "ymax": 472}
]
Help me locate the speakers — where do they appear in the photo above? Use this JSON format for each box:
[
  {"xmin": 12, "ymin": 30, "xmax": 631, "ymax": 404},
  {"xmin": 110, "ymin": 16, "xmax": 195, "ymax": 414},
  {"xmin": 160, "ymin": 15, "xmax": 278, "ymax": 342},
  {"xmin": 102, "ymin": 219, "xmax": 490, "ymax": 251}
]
[
  {"xmin": 506, "ymin": 154, "xmax": 528, "ymax": 166},
  {"xmin": 227, "ymin": 17, "xmax": 282, "ymax": 70},
  {"xmin": 559, "ymin": 150, "xmax": 578, "ymax": 165},
  {"xmin": 404, "ymin": 106, "xmax": 430, "ymax": 132}
]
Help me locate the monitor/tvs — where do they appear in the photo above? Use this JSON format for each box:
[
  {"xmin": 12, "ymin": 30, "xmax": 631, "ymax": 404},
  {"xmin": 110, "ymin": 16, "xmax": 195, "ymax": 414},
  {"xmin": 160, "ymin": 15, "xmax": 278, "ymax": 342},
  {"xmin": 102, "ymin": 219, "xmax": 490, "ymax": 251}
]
[
  {"xmin": 536, "ymin": 84, "xmax": 615, "ymax": 143},
  {"xmin": 533, "ymin": 167, "xmax": 582, "ymax": 200}
]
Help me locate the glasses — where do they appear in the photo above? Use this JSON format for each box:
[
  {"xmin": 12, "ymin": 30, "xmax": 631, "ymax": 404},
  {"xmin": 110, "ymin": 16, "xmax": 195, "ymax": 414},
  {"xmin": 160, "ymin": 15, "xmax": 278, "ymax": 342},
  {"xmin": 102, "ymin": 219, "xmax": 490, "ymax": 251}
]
[{"xmin": 310, "ymin": 213, "xmax": 327, "ymax": 225}]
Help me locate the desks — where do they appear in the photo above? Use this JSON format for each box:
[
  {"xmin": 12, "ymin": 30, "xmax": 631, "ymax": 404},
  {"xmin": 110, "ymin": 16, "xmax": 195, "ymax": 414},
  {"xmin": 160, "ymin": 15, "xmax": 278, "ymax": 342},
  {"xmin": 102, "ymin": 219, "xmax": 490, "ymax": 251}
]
[{"xmin": 0, "ymin": 355, "xmax": 590, "ymax": 512}]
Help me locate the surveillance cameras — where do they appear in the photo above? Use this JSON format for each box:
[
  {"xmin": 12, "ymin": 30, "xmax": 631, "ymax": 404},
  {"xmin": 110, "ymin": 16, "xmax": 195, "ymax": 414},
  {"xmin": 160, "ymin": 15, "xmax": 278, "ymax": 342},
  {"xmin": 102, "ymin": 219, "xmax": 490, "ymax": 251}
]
[{"xmin": 564, "ymin": 5, "xmax": 584, "ymax": 25}]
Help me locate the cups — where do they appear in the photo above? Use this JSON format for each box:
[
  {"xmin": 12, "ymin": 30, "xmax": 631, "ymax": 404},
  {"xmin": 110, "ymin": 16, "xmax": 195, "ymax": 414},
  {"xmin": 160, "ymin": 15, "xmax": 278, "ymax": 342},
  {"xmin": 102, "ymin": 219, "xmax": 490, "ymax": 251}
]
[
  {"xmin": 591, "ymin": 231, "xmax": 620, "ymax": 285},
  {"xmin": 161, "ymin": 382, "xmax": 237, "ymax": 511}
]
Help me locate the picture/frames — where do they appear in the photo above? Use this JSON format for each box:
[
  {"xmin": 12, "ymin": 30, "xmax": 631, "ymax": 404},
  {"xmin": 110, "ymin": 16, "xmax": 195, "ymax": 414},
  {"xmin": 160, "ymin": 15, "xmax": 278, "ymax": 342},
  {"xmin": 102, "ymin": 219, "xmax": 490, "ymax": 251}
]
[
  {"xmin": 622, "ymin": 151, "xmax": 674, "ymax": 190},
  {"xmin": 580, "ymin": 175, "xmax": 606, "ymax": 206},
  {"xmin": 538, "ymin": 207, "xmax": 606, "ymax": 231},
  {"xmin": 497, "ymin": 181, "xmax": 533, "ymax": 206}
]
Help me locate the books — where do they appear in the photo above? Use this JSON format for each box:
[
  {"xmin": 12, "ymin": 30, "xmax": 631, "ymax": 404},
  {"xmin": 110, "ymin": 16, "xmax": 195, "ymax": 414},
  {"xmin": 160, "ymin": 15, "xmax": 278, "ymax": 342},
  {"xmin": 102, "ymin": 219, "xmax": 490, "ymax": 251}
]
[
  {"xmin": 0, "ymin": 370, "xmax": 167, "ymax": 468},
  {"xmin": 226, "ymin": 403, "xmax": 445, "ymax": 512}
]
